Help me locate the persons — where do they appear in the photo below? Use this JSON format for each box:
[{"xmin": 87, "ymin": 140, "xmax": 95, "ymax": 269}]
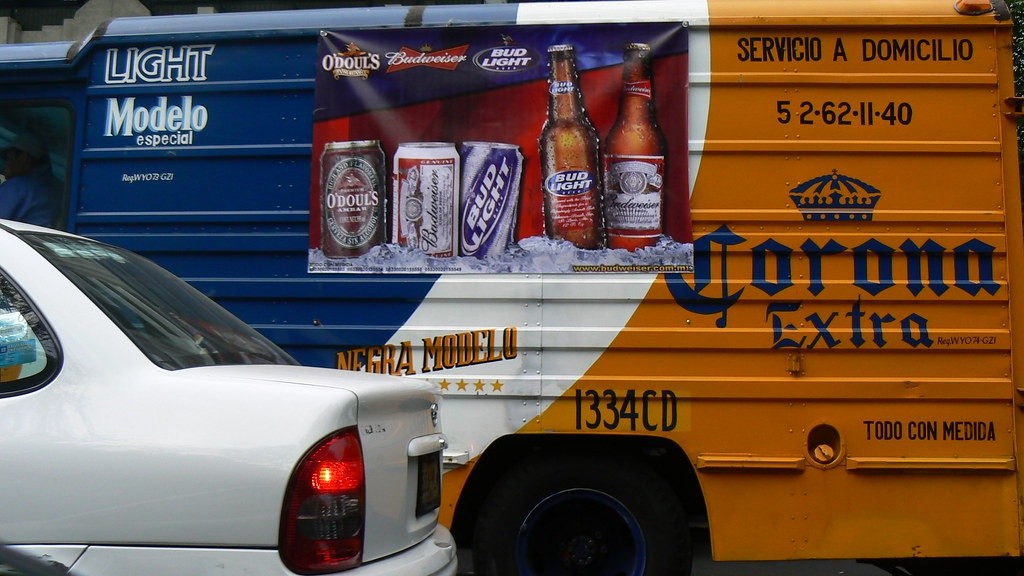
[{"xmin": 0, "ymin": 136, "xmax": 57, "ymax": 227}]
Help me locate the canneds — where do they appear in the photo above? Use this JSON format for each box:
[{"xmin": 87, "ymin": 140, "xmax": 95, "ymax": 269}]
[
  {"xmin": 319, "ymin": 140, "xmax": 388, "ymax": 260},
  {"xmin": 455, "ymin": 141, "xmax": 524, "ymax": 260},
  {"xmin": 390, "ymin": 141, "xmax": 461, "ymax": 260}
]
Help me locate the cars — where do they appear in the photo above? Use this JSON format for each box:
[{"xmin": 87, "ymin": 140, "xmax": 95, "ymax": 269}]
[{"xmin": 0, "ymin": 219, "xmax": 459, "ymax": 575}]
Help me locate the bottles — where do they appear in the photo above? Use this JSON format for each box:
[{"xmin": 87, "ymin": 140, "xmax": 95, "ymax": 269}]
[
  {"xmin": 602, "ymin": 43, "xmax": 667, "ymax": 252},
  {"xmin": 539, "ymin": 44, "xmax": 605, "ymax": 251}
]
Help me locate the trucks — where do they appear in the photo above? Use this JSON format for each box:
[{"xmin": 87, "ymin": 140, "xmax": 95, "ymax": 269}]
[{"xmin": 0, "ymin": 1, "xmax": 1024, "ymax": 576}]
